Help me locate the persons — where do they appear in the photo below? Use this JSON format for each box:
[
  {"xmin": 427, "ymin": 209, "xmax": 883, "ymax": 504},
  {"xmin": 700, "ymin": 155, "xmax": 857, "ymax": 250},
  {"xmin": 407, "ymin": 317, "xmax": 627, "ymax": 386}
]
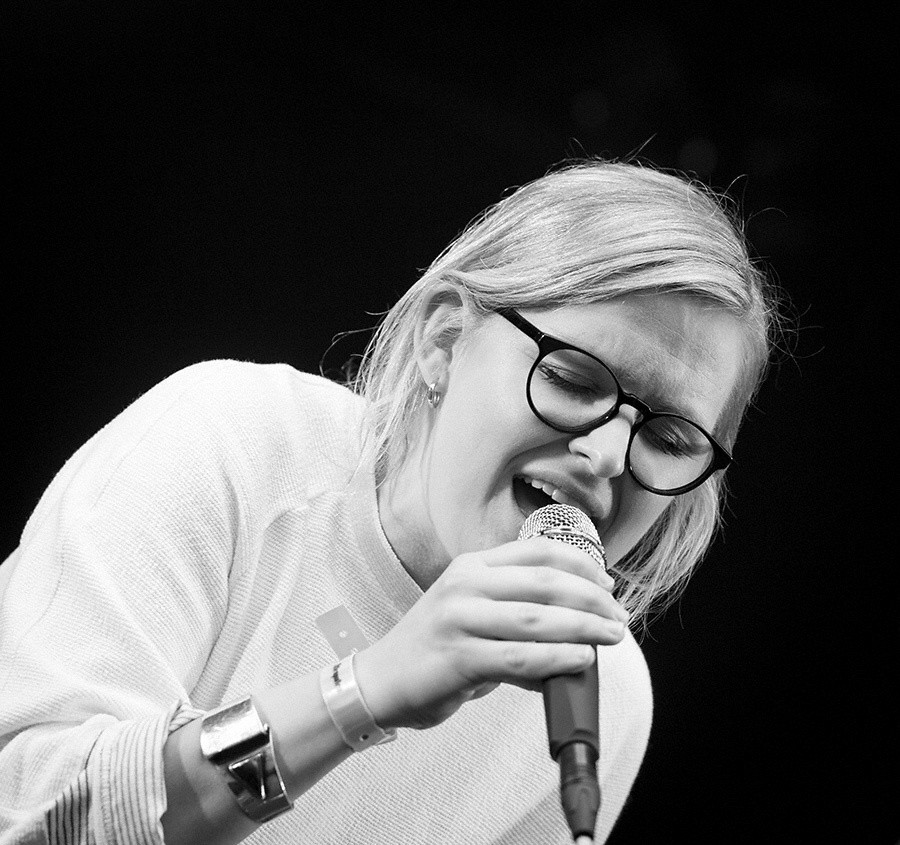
[{"xmin": 0, "ymin": 157, "xmax": 791, "ymax": 845}]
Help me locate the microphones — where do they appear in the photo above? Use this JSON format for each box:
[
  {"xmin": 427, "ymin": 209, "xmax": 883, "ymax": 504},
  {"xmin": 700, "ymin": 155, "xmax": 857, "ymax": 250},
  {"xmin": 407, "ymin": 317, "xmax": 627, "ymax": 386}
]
[{"xmin": 514, "ymin": 501, "xmax": 608, "ymax": 838}]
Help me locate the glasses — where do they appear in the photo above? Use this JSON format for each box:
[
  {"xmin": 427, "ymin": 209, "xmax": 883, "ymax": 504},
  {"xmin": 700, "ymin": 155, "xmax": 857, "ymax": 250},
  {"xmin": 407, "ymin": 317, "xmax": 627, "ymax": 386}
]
[{"xmin": 492, "ymin": 306, "xmax": 734, "ymax": 497}]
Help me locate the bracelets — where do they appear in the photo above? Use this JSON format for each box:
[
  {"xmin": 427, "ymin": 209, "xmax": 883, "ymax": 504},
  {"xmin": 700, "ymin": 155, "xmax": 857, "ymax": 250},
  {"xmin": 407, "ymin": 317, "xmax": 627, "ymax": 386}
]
[
  {"xmin": 314, "ymin": 603, "xmax": 398, "ymax": 751},
  {"xmin": 199, "ymin": 692, "xmax": 295, "ymax": 824}
]
